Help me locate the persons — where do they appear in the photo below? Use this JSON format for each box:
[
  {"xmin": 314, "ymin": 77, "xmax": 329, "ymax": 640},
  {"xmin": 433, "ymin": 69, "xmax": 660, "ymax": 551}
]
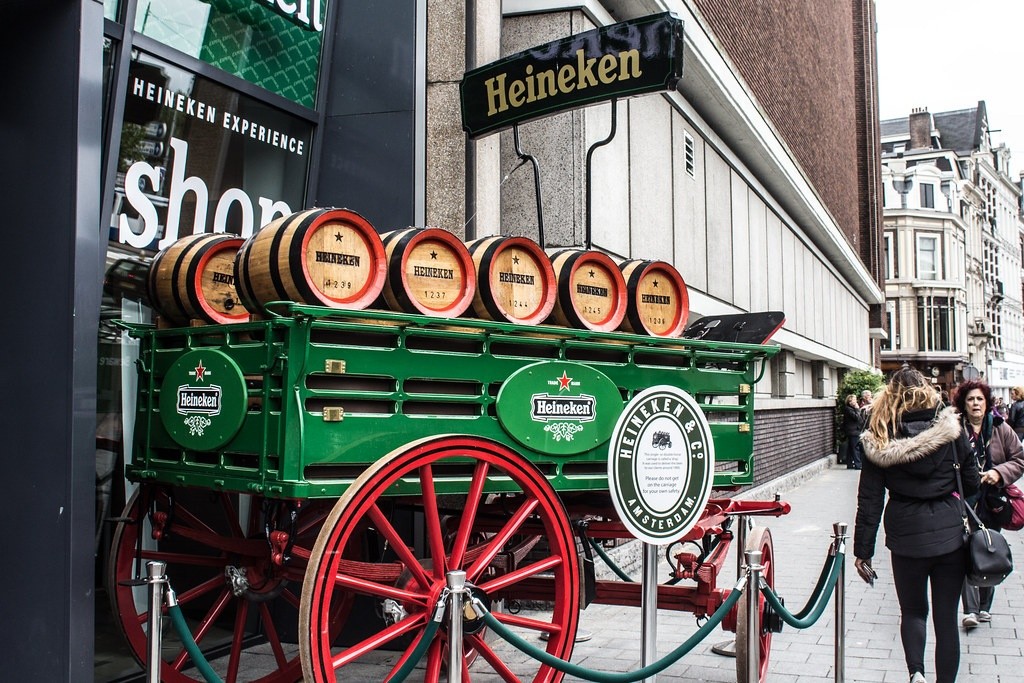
[
  {"xmin": 933, "ymin": 382, "xmax": 1024, "ymax": 445},
  {"xmin": 838, "ymin": 387, "xmax": 873, "ymax": 470},
  {"xmin": 853, "ymin": 367, "xmax": 981, "ymax": 683},
  {"xmin": 954, "ymin": 381, "xmax": 1024, "ymax": 626}
]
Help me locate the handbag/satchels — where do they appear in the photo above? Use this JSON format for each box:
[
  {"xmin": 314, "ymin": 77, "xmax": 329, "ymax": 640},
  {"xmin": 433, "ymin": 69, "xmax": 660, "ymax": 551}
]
[
  {"xmin": 1002, "ymin": 483, "xmax": 1024, "ymax": 530},
  {"xmin": 962, "ymin": 527, "xmax": 1014, "ymax": 588}
]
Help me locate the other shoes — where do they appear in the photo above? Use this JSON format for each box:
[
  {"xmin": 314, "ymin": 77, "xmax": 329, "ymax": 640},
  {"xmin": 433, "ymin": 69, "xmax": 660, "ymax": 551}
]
[
  {"xmin": 978, "ymin": 610, "xmax": 993, "ymax": 622},
  {"xmin": 910, "ymin": 671, "xmax": 927, "ymax": 683},
  {"xmin": 961, "ymin": 612, "xmax": 979, "ymax": 629}
]
